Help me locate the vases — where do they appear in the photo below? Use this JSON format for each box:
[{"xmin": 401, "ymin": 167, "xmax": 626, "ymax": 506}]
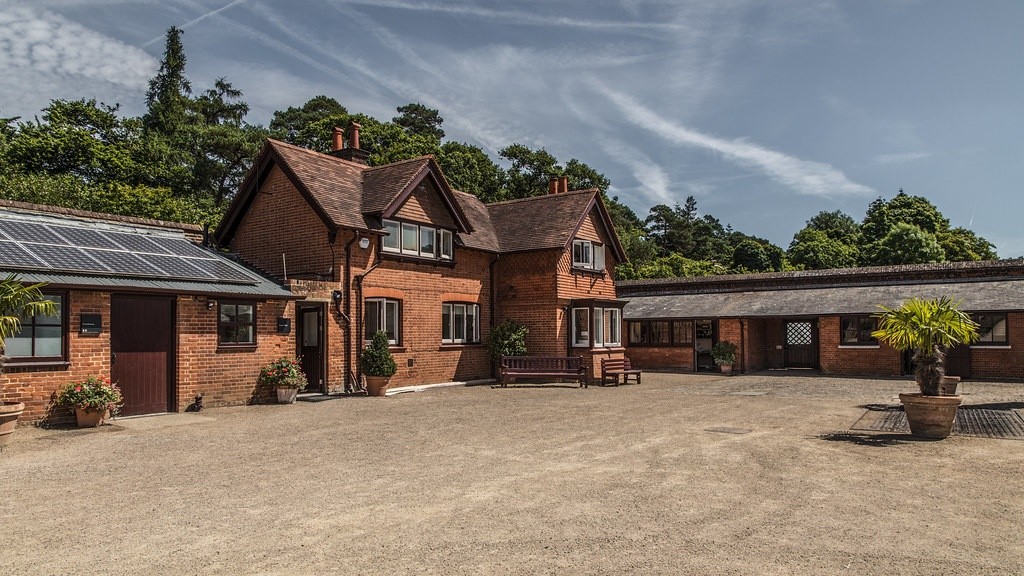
[
  {"xmin": 75, "ymin": 408, "xmax": 105, "ymax": 427},
  {"xmin": 275, "ymin": 385, "xmax": 298, "ymax": 404}
]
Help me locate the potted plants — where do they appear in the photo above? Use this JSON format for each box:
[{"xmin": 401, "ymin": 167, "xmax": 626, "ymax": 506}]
[
  {"xmin": 871, "ymin": 289, "xmax": 982, "ymax": 437},
  {"xmin": 0, "ymin": 266, "xmax": 63, "ymax": 450},
  {"xmin": 358, "ymin": 330, "xmax": 398, "ymax": 396},
  {"xmin": 481, "ymin": 317, "xmax": 530, "ymax": 383},
  {"xmin": 708, "ymin": 340, "xmax": 740, "ymax": 374}
]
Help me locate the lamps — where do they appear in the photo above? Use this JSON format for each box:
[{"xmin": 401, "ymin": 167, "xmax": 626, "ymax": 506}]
[{"xmin": 206, "ymin": 302, "xmax": 213, "ymax": 310}]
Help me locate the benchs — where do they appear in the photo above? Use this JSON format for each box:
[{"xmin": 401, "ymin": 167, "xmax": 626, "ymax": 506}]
[
  {"xmin": 601, "ymin": 356, "xmax": 642, "ymax": 387},
  {"xmin": 500, "ymin": 354, "xmax": 589, "ymax": 388}
]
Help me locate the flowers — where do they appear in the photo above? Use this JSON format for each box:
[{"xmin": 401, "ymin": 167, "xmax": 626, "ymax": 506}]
[
  {"xmin": 259, "ymin": 354, "xmax": 309, "ymax": 393},
  {"xmin": 55, "ymin": 378, "xmax": 124, "ymax": 422}
]
